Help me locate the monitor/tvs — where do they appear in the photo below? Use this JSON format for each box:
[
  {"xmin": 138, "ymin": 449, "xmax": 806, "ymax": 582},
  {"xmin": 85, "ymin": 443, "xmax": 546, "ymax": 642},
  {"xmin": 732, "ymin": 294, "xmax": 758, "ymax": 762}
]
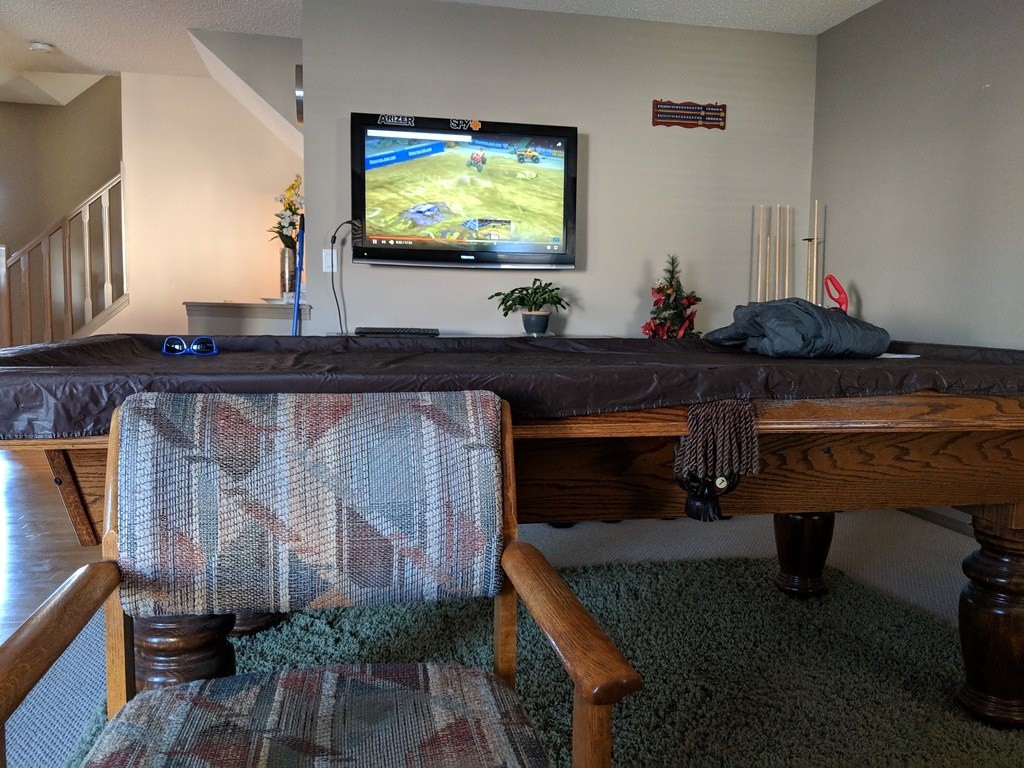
[{"xmin": 349, "ymin": 112, "xmax": 578, "ymax": 269}]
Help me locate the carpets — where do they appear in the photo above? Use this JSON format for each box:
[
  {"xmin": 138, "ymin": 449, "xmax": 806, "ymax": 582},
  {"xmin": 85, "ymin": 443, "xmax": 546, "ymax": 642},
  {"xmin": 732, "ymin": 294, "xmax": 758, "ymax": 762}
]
[{"xmin": 61, "ymin": 557, "xmax": 1024, "ymax": 768}]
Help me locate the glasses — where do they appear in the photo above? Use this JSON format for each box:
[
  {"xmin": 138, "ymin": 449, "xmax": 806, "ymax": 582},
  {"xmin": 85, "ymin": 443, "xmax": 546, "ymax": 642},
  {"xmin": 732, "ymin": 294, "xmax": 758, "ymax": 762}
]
[{"xmin": 161, "ymin": 336, "xmax": 219, "ymax": 356}]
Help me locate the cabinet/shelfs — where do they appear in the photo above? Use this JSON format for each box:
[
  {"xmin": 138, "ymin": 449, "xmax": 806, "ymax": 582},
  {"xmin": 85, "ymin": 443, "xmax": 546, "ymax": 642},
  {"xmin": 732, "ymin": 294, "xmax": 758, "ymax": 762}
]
[{"xmin": 183, "ymin": 302, "xmax": 311, "ymax": 335}]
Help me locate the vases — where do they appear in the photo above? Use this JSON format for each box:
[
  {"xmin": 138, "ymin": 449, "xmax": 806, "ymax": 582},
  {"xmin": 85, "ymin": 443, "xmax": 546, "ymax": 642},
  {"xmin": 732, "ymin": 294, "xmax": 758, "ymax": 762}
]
[{"xmin": 277, "ymin": 248, "xmax": 295, "ymax": 299}]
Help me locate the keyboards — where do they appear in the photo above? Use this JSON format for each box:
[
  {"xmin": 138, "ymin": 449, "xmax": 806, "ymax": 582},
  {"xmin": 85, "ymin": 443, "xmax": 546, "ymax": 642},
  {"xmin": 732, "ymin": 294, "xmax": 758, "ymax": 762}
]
[{"xmin": 355, "ymin": 327, "xmax": 439, "ymax": 336}]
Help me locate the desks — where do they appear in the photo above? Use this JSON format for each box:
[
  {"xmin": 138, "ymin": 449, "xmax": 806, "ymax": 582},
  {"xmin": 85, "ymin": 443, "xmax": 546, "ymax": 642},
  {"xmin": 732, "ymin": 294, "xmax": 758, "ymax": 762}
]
[
  {"xmin": 325, "ymin": 331, "xmax": 616, "ymax": 338},
  {"xmin": 0, "ymin": 332, "xmax": 1024, "ymax": 734}
]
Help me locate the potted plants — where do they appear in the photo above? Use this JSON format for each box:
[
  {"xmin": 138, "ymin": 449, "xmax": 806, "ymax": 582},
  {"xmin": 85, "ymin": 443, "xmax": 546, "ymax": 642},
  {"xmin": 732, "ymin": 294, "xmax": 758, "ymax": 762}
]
[{"xmin": 489, "ymin": 278, "xmax": 572, "ymax": 333}]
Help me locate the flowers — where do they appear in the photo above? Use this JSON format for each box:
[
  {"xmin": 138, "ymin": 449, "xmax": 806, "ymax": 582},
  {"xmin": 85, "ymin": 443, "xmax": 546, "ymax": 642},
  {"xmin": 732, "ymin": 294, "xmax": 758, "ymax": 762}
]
[{"xmin": 265, "ymin": 173, "xmax": 304, "ymax": 251}]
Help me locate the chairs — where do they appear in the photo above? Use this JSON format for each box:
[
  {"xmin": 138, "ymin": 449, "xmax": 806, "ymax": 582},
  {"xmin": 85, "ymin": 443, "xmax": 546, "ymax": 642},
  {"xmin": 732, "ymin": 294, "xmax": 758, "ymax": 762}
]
[{"xmin": 0, "ymin": 392, "xmax": 642, "ymax": 768}]
